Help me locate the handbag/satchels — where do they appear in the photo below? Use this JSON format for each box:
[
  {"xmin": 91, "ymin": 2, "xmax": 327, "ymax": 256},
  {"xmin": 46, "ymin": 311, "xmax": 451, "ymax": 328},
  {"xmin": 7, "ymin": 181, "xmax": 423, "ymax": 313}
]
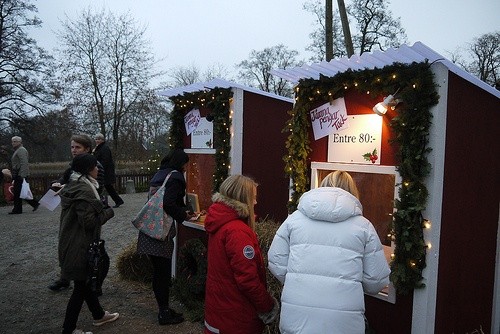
[
  {"xmin": 86, "ymin": 241, "xmax": 109, "ymax": 296},
  {"xmin": 20, "ymin": 177, "xmax": 33, "ymax": 200},
  {"xmin": 131, "ymin": 170, "xmax": 179, "ymax": 242}
]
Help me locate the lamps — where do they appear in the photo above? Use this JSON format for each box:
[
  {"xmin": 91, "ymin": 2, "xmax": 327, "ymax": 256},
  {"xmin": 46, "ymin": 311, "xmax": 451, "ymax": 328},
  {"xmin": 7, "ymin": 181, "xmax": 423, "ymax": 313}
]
[{"xmin": 372, "ymin": 95, "xmax": 403, "ymax": 116}]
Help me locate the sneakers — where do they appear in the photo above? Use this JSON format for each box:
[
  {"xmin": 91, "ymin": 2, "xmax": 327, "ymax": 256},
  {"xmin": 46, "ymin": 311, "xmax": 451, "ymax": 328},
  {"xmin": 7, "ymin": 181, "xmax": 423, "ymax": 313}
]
[
  {"xmin": 93, "ymin": 311, "xmax": 120, "ymax": 326},
  {"xmin": 62, "ymin": 328, "xmax": 92, "ymax": 334}
]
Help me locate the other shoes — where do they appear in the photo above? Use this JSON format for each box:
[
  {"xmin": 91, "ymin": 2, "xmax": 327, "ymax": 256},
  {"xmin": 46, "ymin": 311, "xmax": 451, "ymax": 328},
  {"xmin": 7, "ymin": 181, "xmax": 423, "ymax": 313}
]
[
  {"xmin": 33, "ymin": 203, "xmax": 40, "ymax": 211},
  {"xmin": 47, "ymin": 278, "xmax": 68, "ymax": 291},
  {"xmin": 8, "ymin": 212, "xmax": 22, "ymax": 214},
  {"xmin": 113, "ymin": 201, "xmax": 124, "ymax": 208},
  {"xmin": 158, "ymin": 309, "xmax": 184, "ymax": 325}
]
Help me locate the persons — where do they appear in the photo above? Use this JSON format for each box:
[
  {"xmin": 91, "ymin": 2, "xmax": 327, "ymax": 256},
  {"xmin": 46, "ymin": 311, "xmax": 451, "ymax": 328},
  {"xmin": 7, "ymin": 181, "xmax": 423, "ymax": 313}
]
[
  {"xmin": 58, "ymin": 152, "xmax": 119, "ymax": 334},
  {"xmin": 266, "ymin": 170, "xmax": 391, "ymax": 334},
  {"xmin": 204, "ymin": 174, "xmax": 279, "ymax": 334},
  {"xmin": 137, "ymin": 148, "xmax": 197, "ymax": 325},
  {"xmin": 47, "ymin": 134, "xmax": 105, "ymax": 290},
  {"xmin": 91, "ymin": 132, "xmax": 124, "ymax": 207},
  {"xmin": 8, "ymin": 136, "xmax": 40, "ymax": 214}
]
